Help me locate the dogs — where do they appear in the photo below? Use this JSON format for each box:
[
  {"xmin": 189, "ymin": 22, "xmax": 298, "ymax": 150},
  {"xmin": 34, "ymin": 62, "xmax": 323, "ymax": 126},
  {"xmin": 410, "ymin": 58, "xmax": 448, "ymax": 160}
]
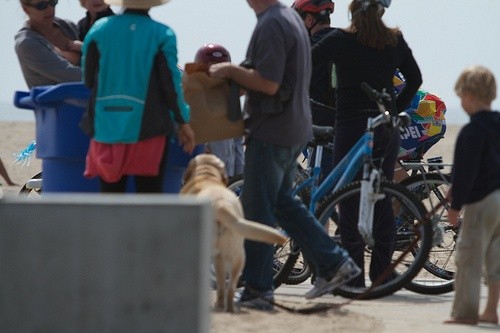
[{"xmin": 178, "ymin": 153, "xmax": 287, "ymax": 313}]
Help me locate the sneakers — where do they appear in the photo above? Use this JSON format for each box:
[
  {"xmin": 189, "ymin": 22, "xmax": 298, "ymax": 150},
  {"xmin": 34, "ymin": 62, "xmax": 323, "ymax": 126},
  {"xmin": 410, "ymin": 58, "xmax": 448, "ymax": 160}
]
[
  {"xmin": 305, "ymin": 258, "xmax": 362, "ymax": 299},
  {"xmin": 234, "ymin": 287, "xmax": 274, "ymax": 310}
]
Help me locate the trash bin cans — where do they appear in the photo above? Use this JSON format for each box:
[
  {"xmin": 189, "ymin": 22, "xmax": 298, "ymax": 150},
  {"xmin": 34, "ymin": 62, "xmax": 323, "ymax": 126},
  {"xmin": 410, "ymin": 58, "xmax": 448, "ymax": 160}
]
[{"xmin": 13, "ymin": 82, "xmax": 136, "ymax": 193}]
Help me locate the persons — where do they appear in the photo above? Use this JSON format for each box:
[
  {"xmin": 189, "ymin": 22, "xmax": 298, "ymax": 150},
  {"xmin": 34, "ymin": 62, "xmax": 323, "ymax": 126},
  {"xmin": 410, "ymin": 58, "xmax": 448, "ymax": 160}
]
[
  {"xmin": 442, "ymin": 67, "xmax": 500, "ymax": 325},
  {"xmin": 81, "ymin": 0, "xmax": 195, "ymax": 194},
  {"xmin": 14, "ymin": 0, "xmax": 447, "ymax": 247},
  {"xmin": 0, "ymin": 160, "xmax": 20, "ymax": 187},
  {"xmin": 310, "ymin": 0, "xmax": 422, "ymax": 288},
  {"xmin": 209, "ymin": 0, "xmax": 361, "ymax": 311}
]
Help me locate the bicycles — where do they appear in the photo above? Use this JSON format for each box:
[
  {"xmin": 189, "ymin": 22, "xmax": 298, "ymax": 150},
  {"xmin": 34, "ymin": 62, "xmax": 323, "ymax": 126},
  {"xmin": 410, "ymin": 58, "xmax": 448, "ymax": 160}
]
[{"xmin": 210, "ymin": 83, "xmax": 465, "ymax": 299}]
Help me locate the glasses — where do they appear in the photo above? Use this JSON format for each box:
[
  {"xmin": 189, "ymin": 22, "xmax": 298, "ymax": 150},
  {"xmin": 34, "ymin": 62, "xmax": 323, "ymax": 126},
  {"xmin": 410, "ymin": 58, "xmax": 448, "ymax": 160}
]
[{"xmin": 26, "ymin": 0, "xmax": 58, "ymax": 11}]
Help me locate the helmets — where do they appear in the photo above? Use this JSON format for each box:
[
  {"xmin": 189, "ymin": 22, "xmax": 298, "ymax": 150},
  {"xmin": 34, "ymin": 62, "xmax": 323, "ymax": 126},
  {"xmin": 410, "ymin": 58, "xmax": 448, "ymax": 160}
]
[
  {"xmin": 290, "ymin": 0, "xmax": 335, "ymax": 15},
  {"xmin": 193, "ymin": 44, "xmax": 232, "ymax": 63}
]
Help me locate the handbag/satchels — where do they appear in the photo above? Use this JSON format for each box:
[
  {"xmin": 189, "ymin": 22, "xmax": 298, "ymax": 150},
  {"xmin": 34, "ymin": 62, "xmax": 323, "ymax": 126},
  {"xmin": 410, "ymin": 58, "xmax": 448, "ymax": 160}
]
[{"xmin": 181, "ymin": 62, "xmax": 247, "ymax": 145}]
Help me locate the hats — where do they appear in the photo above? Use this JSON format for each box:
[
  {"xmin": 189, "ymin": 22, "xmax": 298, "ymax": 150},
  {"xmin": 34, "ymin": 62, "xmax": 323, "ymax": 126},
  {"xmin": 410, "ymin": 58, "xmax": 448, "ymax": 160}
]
[{"xmin": 103, "ymin": 0, "xmax": 171, "ymax": 8}]
